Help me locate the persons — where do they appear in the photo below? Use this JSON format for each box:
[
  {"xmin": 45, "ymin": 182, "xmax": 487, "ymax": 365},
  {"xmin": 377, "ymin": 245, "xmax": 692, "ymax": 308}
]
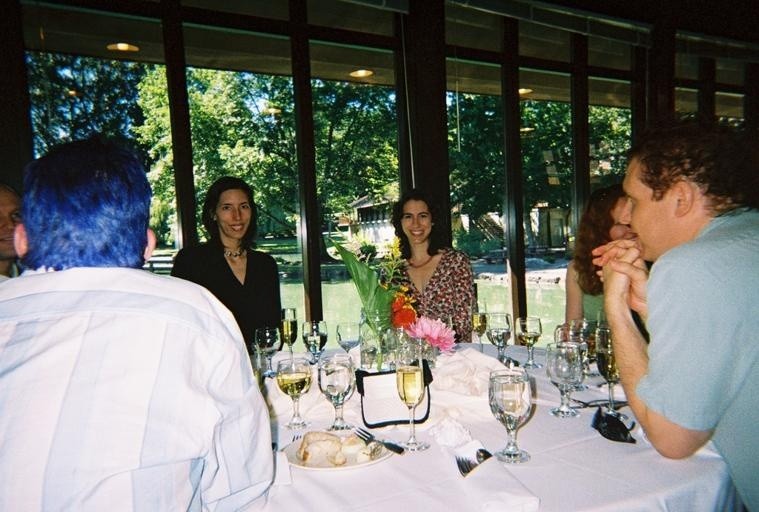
[
  {"xmin": 171, "ymin": 177, "xmax": 284, "ymax": 352},
  {"xmin": 379, "ymin": 190, "xmax": 475, "ymax": 342},
  {"xmin": 2, "ymin": 134, "xmax": 279, "ymax": 512},
  {"xmin": 565, "ymin": 181, "xmax": 633, "ymax": 350},
  {"xmin": 2, "ymin": 178, "xmax": 26, "ymax": 280},
  {"xmin": 592, "ymin": 112, "xmax": 758, "ymax": 512}
]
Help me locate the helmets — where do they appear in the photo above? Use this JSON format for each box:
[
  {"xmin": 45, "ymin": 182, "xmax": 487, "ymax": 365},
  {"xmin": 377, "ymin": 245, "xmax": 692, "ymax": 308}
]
[{"xmin": 280, "ymin": 430, "xmax": 395, "ymax": 472}]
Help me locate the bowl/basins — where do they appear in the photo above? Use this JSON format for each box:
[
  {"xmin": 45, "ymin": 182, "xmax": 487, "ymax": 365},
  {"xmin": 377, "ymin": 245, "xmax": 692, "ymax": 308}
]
[
  {"xmin": 407, "ymin": 254, "xmax": 433, "ymax": 269},
  {"xmin": 224, "ymin": 245, "xmax": 244, "ymax": 258}
]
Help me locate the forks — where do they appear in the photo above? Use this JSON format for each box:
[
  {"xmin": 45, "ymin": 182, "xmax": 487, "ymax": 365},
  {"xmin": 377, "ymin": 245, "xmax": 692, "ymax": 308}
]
[
  {"xmin": 453, "ymin": 456, "xmax": 474, "ymax": 477},
  {"xmin": 354, "ymin": 427, "xmax": 404, "ymax": 454}
]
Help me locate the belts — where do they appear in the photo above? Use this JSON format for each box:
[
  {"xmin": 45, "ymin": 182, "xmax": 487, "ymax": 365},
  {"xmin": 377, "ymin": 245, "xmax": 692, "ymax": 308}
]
[
  {"xmin": 546, "ymin": 319, "xmax": 628, "ymax": 422},
  {"xmin": 276, "ymin": 357, "xmax": 313, "ymax": 431},
  {"xmin": 471, "ymin": 312, "xmax": 543, "ymax": 370},
  {"xmin": 393, "ymin": 344, "xmax": 431, "ymax": 452},
  {"xmin": 317, "ymin": 354, "xmax": 353, "ymax": 433},
  {"xmin": 251, "ymin": 307, "xmax": 297, "ymax": 381},
  {"xmin": 301, "ymin": 319, "xmax": 328, "ymax": 366},
  {"xmin": 489, "ymin": 369, "xmax": 533, "ymax": 464},
  {"xmin": 335, "ymin": 322, "xmax": 359, "ymax": 356}
]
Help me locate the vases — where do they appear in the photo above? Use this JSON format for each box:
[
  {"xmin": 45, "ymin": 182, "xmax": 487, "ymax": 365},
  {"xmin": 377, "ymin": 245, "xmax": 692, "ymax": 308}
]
[{"xmin": 331, "ymin": 215, "xmax": 457, "ymax": 372}]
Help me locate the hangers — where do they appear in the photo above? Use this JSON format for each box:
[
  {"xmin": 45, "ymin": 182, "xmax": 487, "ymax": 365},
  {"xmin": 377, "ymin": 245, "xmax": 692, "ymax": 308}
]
[{"xmin": 476, "ymin": 449, "xmax": 492, "ymax": 464}]
